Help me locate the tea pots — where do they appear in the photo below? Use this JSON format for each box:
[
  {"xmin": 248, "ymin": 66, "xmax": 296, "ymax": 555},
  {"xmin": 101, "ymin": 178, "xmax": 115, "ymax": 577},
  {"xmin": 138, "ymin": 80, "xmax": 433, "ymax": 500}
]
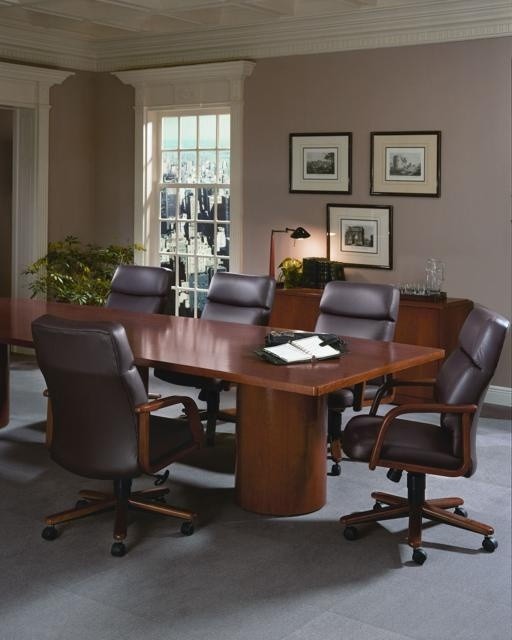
[{"xmin": 424, "ymin": 257, "xmax": 446, "ymax": 295}]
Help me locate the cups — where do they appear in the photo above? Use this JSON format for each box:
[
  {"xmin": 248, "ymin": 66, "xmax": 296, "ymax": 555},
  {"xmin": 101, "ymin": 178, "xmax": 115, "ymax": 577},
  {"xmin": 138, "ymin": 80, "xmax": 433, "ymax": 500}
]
[{"xmin": 397, "ymin": 280, "xmax": 425, "ymax": 296}]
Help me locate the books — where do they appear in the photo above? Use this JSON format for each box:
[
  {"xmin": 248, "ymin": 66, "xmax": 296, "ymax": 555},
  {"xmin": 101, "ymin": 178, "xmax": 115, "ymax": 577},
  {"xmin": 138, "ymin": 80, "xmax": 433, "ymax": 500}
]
[{"xmin": 263, "ymin": 336, "xmax": 341, "ymax": 363}]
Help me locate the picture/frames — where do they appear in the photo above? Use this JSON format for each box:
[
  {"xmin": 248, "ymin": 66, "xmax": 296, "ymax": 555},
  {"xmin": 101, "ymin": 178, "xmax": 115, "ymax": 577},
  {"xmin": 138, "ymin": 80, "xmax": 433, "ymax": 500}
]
[
  {"xmin": 325, "ymin": 202, "xmax": 393, "ymax": 270},
  {"xmin": 369, "ymin": 129, "xmax": 441, "ymax": 198},
  {"xmin": 288, "ymin": 132, "xmax": 352, "ymax": 195}
]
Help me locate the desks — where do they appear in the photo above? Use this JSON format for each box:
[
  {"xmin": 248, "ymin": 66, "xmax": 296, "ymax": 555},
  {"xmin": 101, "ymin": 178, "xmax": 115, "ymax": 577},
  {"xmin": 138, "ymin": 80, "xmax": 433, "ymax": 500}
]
[
  {"xmin": 269, "ymin": 285, "xmax": 475, "ymax": 405},
  {"xmin": 0, "ymin": 297, "xmax": 446, "ymax": 517}
]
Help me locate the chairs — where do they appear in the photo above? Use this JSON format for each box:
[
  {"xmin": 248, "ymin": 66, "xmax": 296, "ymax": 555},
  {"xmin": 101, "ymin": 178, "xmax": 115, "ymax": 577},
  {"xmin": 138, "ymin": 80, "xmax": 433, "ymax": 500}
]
[
  {"xmin": 339, "ymin": 303, "xmax": 510, "ymax": 565},
  {"xmin": 313, "ymin": 280, "xmax": 401, "ymax": 474},
  {"xmin": 154, "ymin": 271, "xmax": 277, "ymax": 448},
  {"xmin": 30, "ymin": 313, "xmax": 205, "ymax": 557},
  {"xmin": 104, "ymin": 264, "xmax": 173, "ymax": 400}
]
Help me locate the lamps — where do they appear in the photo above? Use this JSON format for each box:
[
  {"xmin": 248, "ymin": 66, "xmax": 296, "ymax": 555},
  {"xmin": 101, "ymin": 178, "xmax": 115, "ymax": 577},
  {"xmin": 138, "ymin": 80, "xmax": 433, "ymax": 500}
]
[{"xmin": 269, "ymin": 226, "xmax": 311, "ymax": 289}]
